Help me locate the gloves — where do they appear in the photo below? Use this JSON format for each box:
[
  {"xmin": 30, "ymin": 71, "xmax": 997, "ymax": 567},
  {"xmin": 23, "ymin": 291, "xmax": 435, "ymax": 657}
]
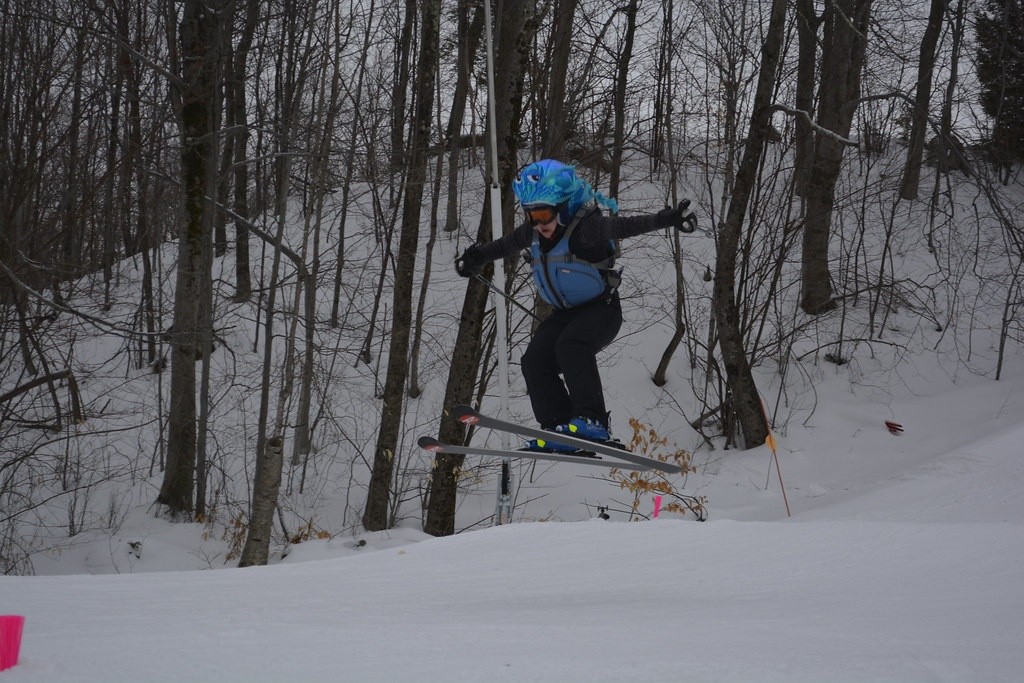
[
  {"xmin": 674, "ymin": 198, "xmax": 698, "ymax": 233},
  {"xmin": 454, "ymin": 243, "xmax": 484, "ymax": 278}
]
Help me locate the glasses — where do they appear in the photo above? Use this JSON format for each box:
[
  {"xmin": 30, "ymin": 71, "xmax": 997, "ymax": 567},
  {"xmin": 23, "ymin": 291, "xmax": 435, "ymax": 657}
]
[{"xmin": 529, "ymin": 214, "xmax": 557, "ymax": 227}]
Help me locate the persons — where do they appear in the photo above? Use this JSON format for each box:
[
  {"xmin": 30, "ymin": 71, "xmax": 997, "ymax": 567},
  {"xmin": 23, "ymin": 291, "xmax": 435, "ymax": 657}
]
[{"xmin": 454, "ymin": 160, "xmax": 699, "ymax": 455}]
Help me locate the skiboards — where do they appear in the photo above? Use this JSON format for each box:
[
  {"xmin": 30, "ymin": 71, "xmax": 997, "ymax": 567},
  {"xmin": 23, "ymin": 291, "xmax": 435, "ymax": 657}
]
[{"xmin": 418, "ymin": 405, "xmax": 683, "ymax": 476}]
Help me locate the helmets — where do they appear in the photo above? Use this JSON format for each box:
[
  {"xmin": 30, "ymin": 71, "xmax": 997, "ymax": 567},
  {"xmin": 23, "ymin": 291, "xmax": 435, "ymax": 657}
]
[{"xmin": 514, "ymin": 159, "xmax": 582, "ymax": 226}]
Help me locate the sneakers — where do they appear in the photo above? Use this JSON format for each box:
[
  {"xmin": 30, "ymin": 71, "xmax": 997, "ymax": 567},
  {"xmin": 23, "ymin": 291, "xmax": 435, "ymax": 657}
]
[
  {"xmin": 555, "ymin": 410, "xmax": 612, "ymax": 441},
  {"xmin": 525, "ymin": 438, "xmax": 584, "ymax": 453}
]
